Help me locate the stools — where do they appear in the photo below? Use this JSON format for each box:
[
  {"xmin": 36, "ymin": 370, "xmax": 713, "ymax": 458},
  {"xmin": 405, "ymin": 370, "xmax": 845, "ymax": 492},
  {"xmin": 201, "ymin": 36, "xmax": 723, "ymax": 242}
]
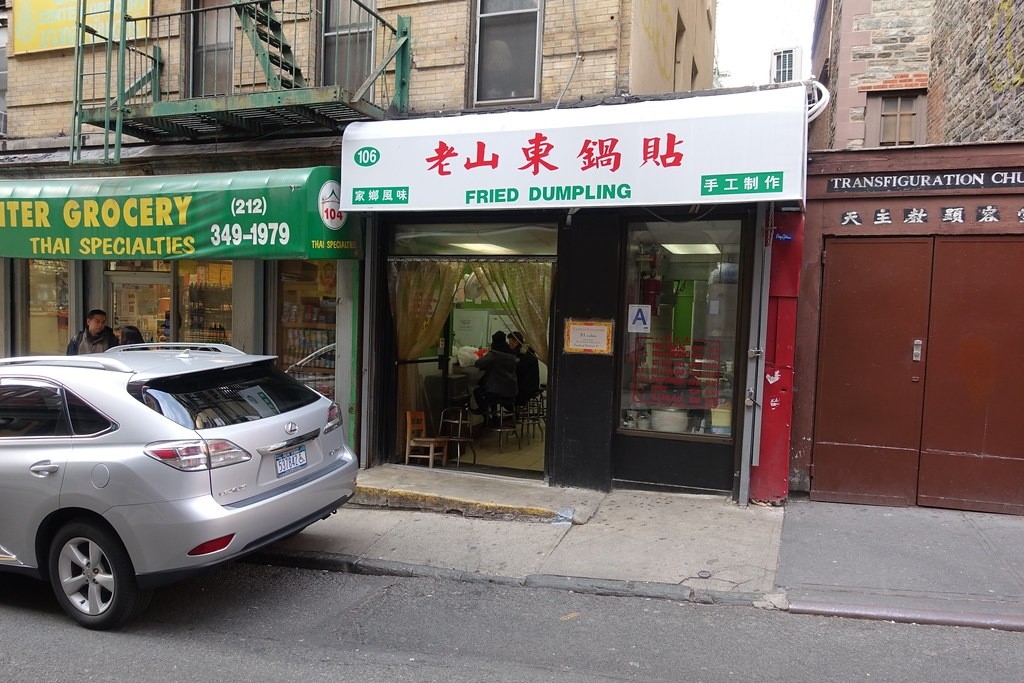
[
  {"xmin": 438, "ymin": 390, "xmax": 475, "ymax": 468},
  {"xmin": 483, "ymin": 383, "xmax": 546, "ymax": 453}
]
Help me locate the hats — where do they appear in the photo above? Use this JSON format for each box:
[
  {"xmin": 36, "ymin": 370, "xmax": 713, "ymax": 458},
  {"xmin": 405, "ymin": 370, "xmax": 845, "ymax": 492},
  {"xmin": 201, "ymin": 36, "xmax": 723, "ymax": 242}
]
[{"xmin": 492, "ymin": 331, "xmax": 507, "ymax": 342}]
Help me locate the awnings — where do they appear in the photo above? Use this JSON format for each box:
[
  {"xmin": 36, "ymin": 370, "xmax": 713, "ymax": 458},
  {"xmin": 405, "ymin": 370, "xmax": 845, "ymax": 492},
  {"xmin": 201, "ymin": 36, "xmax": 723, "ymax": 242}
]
[
  {"xmin": 0, "ymin": 166, "xmax": 356, "ymax": 259},
  {"xmin": 338, "ymin": 86, "xmax": 809, "ymax": 213}
]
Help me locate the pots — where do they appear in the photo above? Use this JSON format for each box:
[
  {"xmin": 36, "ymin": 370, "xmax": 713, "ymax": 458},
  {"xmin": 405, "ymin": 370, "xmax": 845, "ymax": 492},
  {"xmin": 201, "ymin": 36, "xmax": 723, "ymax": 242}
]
[{"xmin": 651, "ymin": 409, "xmax": 689, "ymax": 432}]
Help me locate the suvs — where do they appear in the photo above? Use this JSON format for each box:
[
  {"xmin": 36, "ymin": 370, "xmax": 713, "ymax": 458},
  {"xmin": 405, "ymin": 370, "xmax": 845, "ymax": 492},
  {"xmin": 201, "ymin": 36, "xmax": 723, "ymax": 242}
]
[{"xmin": 0, "ymin": 342, "xmax": 361, "ymax": 633}]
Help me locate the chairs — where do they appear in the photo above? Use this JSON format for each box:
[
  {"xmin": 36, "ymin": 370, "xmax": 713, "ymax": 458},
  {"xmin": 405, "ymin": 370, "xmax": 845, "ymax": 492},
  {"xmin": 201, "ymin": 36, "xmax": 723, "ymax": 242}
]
[{"xmin": 405, "ymin": 411, "xmax": 451, "ymax": 469}]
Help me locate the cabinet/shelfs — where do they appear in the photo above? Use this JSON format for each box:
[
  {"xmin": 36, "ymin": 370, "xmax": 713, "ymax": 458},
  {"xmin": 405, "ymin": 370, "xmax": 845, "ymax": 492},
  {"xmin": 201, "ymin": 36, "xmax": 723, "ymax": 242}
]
[
  {"xmin": 424, "ymin": 373, "xmax": 469, "ymax": 459},
  {"xmin": 280, "ymin": 322, "xmax": 336, "ymax": 401}
]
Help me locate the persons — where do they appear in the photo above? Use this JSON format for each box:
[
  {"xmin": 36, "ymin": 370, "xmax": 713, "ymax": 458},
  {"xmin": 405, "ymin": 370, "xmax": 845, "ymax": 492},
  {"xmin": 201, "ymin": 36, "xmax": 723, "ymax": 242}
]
[
  {"xmin": 121, "ymin": 326, "xmax": 150, "ymax": 351},
  {"xmin": 643, "ymin": 340, "xmax": 712, "ymax": 419},
  {"xmin": 473, "ymin": 331, "xmax": 539, "ymax": 431},
  {"xmin": 66, "ymin": 309, "xmax": 119, "ymax": 356}
]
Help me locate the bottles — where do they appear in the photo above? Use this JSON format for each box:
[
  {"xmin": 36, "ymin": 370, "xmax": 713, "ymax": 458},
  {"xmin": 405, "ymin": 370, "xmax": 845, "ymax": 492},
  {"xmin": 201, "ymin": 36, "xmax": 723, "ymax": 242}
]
[
  {"xmin": 277, "ymin": 304, "xmax": 335, "ymax": 401},
  {"xmin": 178, "ymin": 279, "xmax": 234, "ymax": 349}
]
